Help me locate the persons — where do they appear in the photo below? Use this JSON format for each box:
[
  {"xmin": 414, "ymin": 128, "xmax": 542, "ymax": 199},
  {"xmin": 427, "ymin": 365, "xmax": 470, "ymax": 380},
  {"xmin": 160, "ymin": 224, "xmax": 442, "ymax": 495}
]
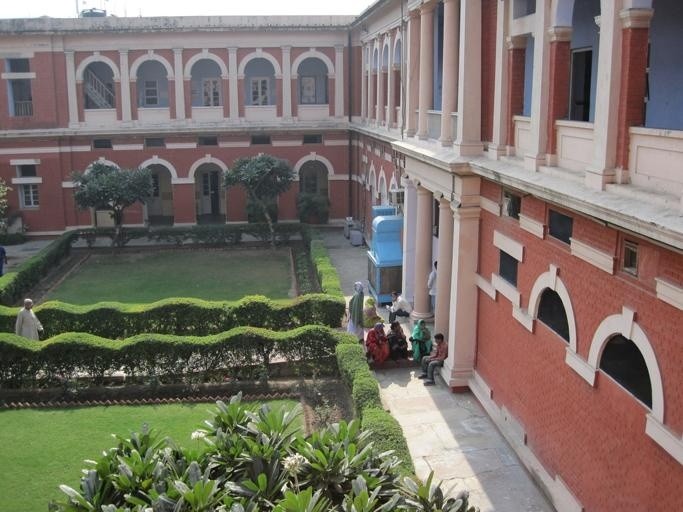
[
  {"xmin": 14, "ymin": 297, "xmax": 44, "ymax": 342},
  {"xmin": 409, "ymin": 319, "xmax": 433, "ymax": 370},
  {"xmin": 0, "ymin": 246, "xmax": 8, "ymax": 277},
  {"xmin": 362, "ymin": 295, "xmax": 385, "ymax": 329},
  {"xmin": 427, "ymin": 261, "xmax": 436, "ymax": 317},
  {"xmin": 386, "ymin": 292, "xmax": 413, "ymax": 324},
  {"xmin": 418, "ymin": 333, "xmax": 449, "ymax": 386},
  {"xmin": 385, "ymin": 322, "xmax": 411, "ymax": 365},
  {"xmin": 347, "ymin": 281, "xmax": 364, "ymax": 344},
  {"xmin": 365, "ymin": 322, "xmax": 390, "ymax": 371}
]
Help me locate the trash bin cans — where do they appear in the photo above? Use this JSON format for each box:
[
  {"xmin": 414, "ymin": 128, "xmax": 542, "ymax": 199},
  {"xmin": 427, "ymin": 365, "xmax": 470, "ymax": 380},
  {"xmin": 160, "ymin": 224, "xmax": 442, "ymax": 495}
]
[{"xmin": 344, "ymin": 220, "xmax": 357, "ymax": 239}]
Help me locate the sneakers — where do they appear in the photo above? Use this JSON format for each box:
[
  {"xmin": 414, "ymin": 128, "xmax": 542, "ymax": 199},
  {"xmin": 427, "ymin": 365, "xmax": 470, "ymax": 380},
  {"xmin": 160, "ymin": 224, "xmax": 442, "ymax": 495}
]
[{"xmin": 418, "ymin": 373, "xmax": 435, "ymax": 385}]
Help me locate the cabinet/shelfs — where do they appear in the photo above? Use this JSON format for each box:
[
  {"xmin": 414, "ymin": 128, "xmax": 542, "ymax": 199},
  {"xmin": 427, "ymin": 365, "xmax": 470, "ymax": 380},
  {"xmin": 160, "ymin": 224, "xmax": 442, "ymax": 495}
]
[{"xmin": 367, "ymin": 252, "xmax": 402, "ymax": 308}]
